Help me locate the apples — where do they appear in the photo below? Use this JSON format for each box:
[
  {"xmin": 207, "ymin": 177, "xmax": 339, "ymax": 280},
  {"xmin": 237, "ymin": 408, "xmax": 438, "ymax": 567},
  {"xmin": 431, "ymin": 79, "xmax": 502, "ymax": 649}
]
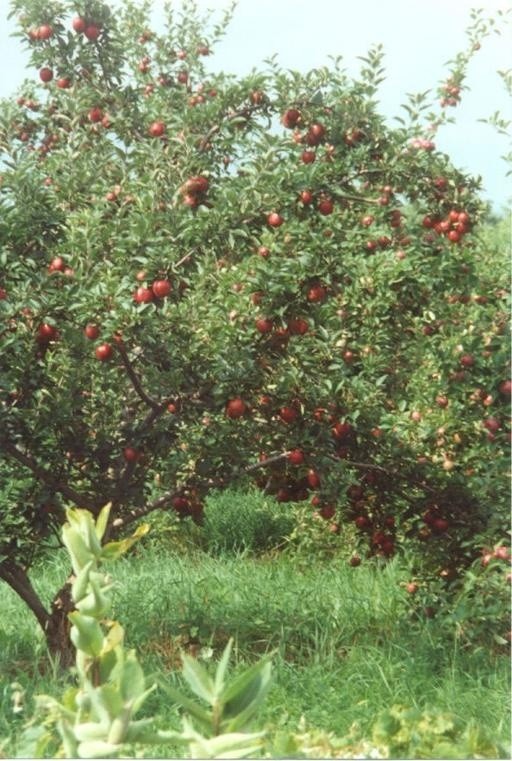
[{"xmin": 0, "ymin": 16, "xmax": 511, "ymax": 595}]
